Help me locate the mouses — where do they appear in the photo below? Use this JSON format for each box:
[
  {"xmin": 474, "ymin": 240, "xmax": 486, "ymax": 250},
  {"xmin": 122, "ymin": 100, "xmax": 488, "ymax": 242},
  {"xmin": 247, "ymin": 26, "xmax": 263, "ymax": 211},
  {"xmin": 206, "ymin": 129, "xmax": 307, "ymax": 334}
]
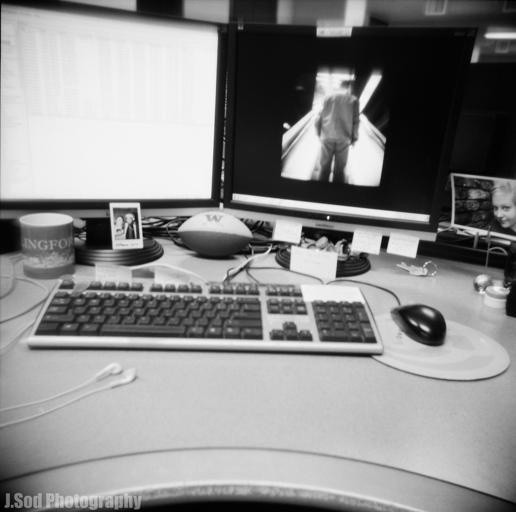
[{"xmin": 392, "ymin": 304, "xmax": 447, "ymax": 345}]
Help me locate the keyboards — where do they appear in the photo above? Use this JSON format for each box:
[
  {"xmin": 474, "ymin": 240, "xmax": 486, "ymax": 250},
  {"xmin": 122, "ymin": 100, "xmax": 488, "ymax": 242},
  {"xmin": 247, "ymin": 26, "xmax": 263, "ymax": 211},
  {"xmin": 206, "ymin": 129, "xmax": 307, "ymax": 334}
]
[{"xmin": 29, "ymin": 279, "xmax": 383, "ymax": 355}]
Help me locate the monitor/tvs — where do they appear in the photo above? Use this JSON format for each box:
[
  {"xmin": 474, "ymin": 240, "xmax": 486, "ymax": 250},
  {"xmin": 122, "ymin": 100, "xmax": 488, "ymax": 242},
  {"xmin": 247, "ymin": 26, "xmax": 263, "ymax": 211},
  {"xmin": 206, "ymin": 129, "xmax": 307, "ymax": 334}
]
[
  {"xmin": 224, "ymin": 21, "xmax": 516, "ymax": 279},
  {"xmin": 0, "ymin": 0, "xmax": 227, "ymax": 267}
]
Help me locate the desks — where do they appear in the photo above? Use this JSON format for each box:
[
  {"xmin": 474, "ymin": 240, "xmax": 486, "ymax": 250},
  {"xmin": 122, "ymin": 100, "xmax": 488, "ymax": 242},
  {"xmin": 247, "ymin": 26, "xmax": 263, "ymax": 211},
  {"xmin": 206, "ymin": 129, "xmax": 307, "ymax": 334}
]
[{"xmin": 0, "ymin": 235, "xmax": 515, "ymax": 509}]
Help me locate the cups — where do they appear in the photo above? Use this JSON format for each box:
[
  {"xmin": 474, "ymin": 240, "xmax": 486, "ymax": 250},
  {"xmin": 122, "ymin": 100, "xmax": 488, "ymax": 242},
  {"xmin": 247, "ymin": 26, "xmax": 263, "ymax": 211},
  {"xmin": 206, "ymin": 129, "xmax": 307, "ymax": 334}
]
[{"xmin": 19, "ymin": 213, "xmax": 74, "ymax": 278}]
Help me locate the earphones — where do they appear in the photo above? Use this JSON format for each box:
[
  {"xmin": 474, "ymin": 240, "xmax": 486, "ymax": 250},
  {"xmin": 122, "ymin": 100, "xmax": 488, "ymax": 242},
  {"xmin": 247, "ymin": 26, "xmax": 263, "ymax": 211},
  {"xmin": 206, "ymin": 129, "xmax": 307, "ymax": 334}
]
[
  {"xmin": 110, "ymin": 368, "xmax": 137, "ymax": 386},
  {"xmin": 96, "ymin": 363, "xmax": 121, "ymax": 377}
]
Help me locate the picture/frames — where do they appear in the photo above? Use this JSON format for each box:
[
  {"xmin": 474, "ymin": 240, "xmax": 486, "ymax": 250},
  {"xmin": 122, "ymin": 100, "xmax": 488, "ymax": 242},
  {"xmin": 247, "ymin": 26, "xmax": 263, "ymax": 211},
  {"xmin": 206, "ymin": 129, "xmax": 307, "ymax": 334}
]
[{"xmin": 449, "ymin": 173, "xmax": 515, "ymax": 240}]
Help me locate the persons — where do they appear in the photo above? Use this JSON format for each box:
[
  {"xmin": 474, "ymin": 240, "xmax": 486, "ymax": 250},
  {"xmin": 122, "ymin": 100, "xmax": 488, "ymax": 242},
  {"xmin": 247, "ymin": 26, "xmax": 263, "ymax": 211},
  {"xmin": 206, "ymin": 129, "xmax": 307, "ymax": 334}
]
[
  {"xmin": 314, "ymin": 80, "xmax": 359, "ymax": 183},
  {"xmin": 492, "ymin": 181, "xmax": 516, "ymax": 233},
  {"xmin": 115, "ymin": 213, "xmax": 137, "ymax": 240}
]
